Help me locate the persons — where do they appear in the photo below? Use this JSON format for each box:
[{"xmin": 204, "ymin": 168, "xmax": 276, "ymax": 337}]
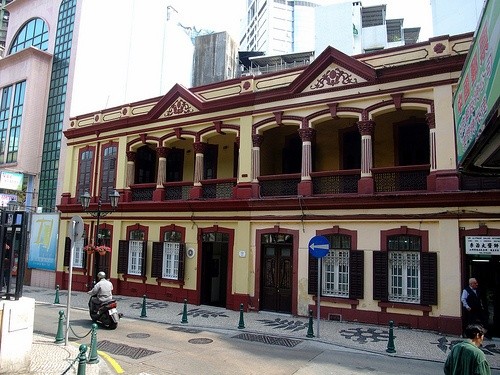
[
  {"xmin": 444, "ymin": 324, "xmax": 491, "ymax": 375},
  {"xmin": 3, "ymin": 243, "xmax": 11, "ymax": 288},
  {"xmin": 88, "ymin": 271, "xmax": 113, "ymax": 315},
  {"xmin": 461, "ymin": 278, "xmax": 481, "ymax": 338}
]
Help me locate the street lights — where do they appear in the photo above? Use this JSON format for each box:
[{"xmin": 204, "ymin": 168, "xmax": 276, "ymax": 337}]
[
  {"xmin": 0, "ymin": 197, "xmax": 17, "ymax": 299},
  {"xmin": 79, "ymin": 189, "xmax": 120, "ymax": 297}
]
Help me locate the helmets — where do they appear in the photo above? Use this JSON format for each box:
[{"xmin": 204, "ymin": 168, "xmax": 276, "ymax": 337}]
[{"xmin": 97, "ymin": 271, "xmax": 105, "ymax": 278}]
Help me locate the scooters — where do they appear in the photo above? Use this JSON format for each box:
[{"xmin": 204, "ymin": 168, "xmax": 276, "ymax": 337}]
[{"xmin": 88, "ymin": 294, "xmax": 123, "ymax": 330}]
